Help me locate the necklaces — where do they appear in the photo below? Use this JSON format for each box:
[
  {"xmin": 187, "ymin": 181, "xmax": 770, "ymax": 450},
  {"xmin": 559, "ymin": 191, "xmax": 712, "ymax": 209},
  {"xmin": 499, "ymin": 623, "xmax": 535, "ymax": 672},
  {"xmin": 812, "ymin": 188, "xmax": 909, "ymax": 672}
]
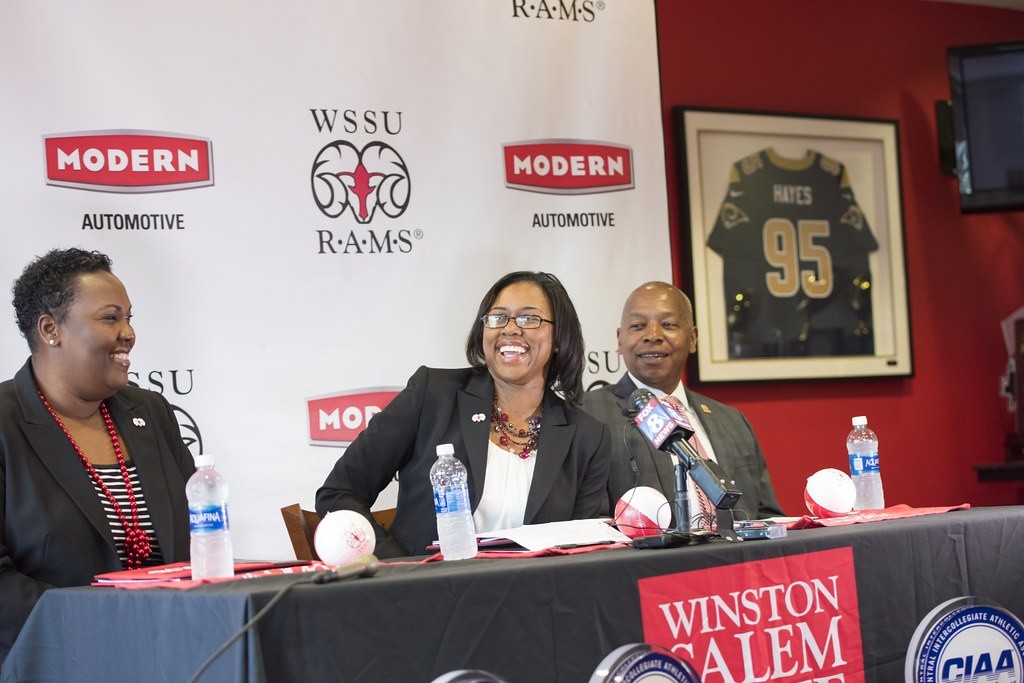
[
  {"xmin": 35, "ymin": 384, "xmax": 152, "ymax": 572},
  {"xmin": 492, "ymin": 391, "xmax": 542, "ymax": 459}
]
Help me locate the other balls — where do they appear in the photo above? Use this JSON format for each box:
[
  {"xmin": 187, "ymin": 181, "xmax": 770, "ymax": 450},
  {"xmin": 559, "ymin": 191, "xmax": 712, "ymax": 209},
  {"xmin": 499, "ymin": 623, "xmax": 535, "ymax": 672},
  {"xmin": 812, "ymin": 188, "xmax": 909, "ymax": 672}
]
[
  {"xmin": 804, "ymin": 468, "xmax": 857, "ymax": 519},
  {"xmin": 614, "ymin": 485, "xmax": 672, "ymax": 539},
  {"xmin": 313, "ymin": 508, "xmax": 376, "ymax": 568}
]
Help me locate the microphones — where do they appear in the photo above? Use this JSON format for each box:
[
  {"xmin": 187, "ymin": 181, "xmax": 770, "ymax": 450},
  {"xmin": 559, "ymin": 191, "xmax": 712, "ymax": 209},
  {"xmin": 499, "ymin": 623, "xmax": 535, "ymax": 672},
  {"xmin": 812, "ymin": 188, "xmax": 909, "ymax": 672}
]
[
  {"xmin": 309, "ymin": 553, "xmax": 379, "ymax": 583},
  {"xmin": 628, "ymin": 388, "xmax": 743, "ymax": 509},
  {"xmin": 622, "ymin": 408, "xmax": 676, "ymax": 524}
]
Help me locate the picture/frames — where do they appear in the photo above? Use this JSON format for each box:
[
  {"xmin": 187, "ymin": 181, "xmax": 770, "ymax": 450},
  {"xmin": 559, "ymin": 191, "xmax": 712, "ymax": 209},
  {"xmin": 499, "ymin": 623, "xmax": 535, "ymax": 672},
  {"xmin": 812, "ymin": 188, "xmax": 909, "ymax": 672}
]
[{"xmin": 671, "ymin": 106, "xmax": 915, "ymax": 385}]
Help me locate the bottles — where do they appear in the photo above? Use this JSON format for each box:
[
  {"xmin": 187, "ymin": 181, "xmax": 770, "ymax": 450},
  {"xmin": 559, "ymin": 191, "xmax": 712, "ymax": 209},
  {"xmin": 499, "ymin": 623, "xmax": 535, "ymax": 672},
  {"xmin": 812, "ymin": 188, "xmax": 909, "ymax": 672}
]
[
  {"xmin": 429, "ymin": 444, "xmax": 478, "ymax": 561},
  {"xmin": 846, "ymin": 415, "xmax": 885, "ymax": 511},
  {"xmin": 185, "ymin": 455, "xmax": 236, "ymax": 581}
]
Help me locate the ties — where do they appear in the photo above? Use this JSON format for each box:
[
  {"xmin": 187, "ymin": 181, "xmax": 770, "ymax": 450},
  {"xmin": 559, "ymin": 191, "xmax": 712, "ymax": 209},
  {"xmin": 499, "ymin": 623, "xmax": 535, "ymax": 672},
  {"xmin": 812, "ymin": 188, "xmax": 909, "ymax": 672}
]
[{"xmin": 660, "ymin": 395, "xmax": 718, "ymax": 527}]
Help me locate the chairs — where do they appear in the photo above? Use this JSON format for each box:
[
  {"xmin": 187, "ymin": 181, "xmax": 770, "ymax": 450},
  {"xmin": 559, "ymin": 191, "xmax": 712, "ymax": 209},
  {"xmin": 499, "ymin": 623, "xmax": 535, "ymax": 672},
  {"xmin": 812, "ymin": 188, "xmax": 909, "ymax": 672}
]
[{"xmin": 282, "ymin": 504, "xmax": 397, "ymax": 562}]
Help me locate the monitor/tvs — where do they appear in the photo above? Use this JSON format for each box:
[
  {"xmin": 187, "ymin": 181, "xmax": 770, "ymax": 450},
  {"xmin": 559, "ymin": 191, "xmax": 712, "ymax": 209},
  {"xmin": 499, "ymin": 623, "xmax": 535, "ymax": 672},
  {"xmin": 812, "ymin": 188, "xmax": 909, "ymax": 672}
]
[{"xmin": 946, "ymin": 39, "xmax": 1024, "ymax": 217}]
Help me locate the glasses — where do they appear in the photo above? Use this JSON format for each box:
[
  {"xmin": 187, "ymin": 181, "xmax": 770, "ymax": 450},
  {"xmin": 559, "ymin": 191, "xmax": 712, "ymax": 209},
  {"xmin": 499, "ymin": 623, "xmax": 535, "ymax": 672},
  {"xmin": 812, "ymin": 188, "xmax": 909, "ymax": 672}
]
[{"xmin": 480, "ymin": 313, "xmax": 556, "ymax": 328}]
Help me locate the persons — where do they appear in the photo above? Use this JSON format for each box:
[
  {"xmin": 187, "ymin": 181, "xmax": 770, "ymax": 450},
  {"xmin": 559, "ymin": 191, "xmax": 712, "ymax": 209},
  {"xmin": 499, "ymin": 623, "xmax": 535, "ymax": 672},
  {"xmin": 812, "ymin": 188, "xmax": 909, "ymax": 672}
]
[
  {"xmin": 314, "ymin": 270, "xmax": 613, "ymax": 561},
  {"xmin": 1, "ymin": 246, "xmax": 200, "ymax": 663},
  {"xmin": 584, "ymin": 280, "xmax": 786, "ymax": 530}
]
